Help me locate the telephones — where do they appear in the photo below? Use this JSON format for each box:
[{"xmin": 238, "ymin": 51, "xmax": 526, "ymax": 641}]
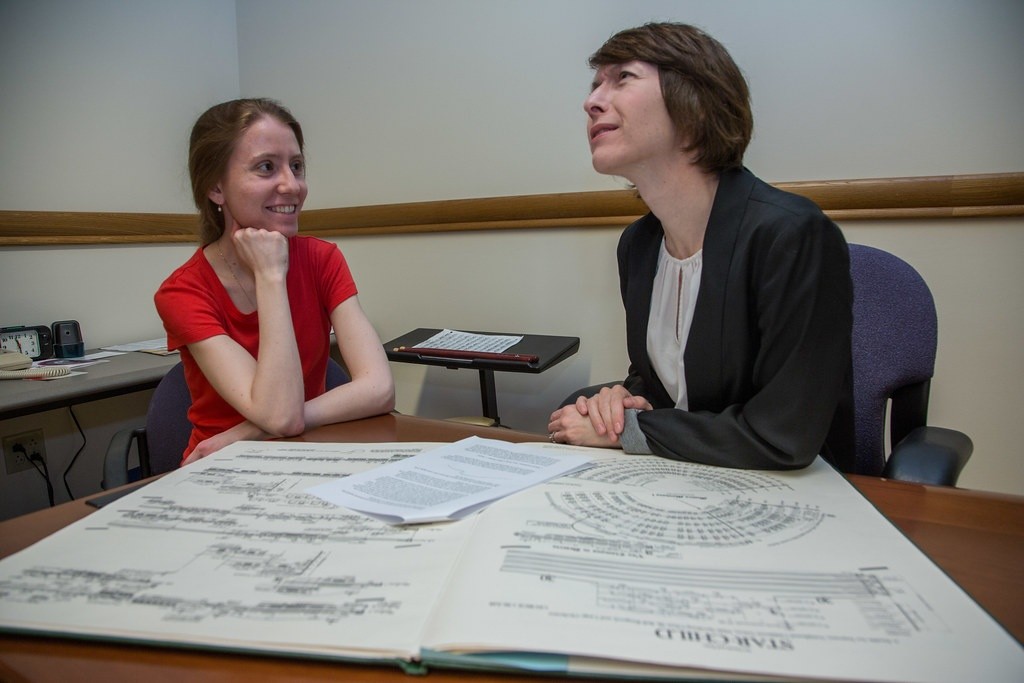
[{"xmin": 0, "ymin": 348, "xmax": 33, "ymax": 370}]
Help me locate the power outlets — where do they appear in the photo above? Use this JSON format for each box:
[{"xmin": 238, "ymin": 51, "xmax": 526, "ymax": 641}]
[{"xmin": 1, "ymin": 428, "xmax": 48, "ymax": 473}]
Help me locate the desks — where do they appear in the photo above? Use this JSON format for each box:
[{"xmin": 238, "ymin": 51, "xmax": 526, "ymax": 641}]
[
  {"xmin": 0, "ymin": 411, "xmax": 1023, "ymax": 683},
  {"xmin": 0, "ymin": 339, "xmax": 183, "ymax": 421}
]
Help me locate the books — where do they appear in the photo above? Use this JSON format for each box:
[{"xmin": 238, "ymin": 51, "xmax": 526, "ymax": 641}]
[{"xmin": 0, "ymin": 439, "xmax": 1024, "ymax": 683}]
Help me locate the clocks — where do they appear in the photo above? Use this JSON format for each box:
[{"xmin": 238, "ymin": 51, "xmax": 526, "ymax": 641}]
[{"xmin": 0, "ymin": 324, "xmax": 55, "ymax": 361}]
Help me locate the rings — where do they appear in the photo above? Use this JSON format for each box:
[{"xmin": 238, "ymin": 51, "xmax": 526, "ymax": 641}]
[{"xmin": 549, "ymin": 432, "xmax": 555, "ymax": 443}]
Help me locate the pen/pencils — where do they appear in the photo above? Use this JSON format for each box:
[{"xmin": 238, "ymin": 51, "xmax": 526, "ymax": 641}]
[{"xmin": 393, "ymin": 347, "xmax": 539, "ymax": 363}]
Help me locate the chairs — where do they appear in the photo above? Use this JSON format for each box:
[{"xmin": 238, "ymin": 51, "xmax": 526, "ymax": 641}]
[
  {"xmin": 144, "ymin": 358, "xmax": 352, "ymax": 476},
  {"xmin": 556, "ymin": 243, "xmax": 974, "ymax": 485}
]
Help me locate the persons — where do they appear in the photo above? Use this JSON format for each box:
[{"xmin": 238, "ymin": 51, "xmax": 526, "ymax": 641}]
[
  {"xmin": 153, "ymin": 98, "xmax": 394, "ymax": 468},
  {"xmin": 549, "ymin": 22, "xmax": 859, "ymax": 472}
]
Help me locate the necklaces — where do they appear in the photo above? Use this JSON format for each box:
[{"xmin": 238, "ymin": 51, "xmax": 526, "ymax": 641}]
[{"xmin": 218, "ymin": 240, "xmax": 256, "ymax": 309}]
